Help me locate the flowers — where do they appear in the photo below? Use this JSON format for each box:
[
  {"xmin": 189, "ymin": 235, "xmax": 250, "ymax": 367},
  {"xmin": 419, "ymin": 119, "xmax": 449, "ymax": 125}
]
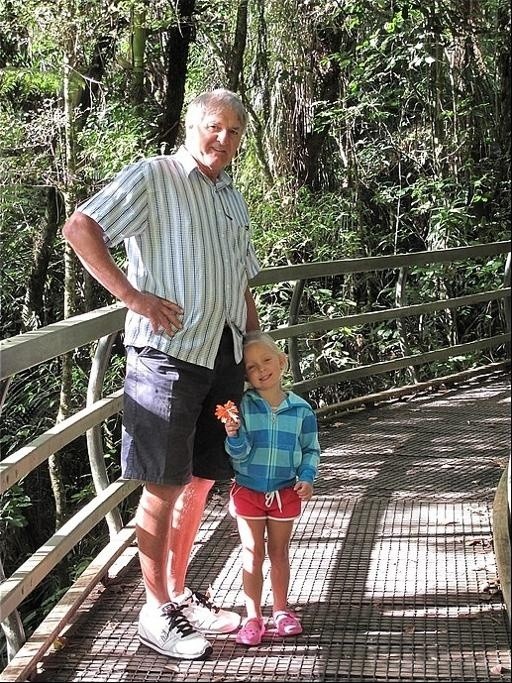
[{"xmin": 215, "ymin": 400, "xmax": 239, "ymax": 424}]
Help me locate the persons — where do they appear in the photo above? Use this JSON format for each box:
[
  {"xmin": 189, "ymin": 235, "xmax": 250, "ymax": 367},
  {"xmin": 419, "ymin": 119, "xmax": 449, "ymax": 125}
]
[
  {"xmin": 213, "ymin": 330, "xmax": 322, "ymax": 645},
  {"xmin": 62, "ymin": 87, "xmax": 265, "ymax": 660}
]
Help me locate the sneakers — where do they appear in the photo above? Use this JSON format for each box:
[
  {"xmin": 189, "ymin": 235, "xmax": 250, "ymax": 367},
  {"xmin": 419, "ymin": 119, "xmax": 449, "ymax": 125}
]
[
  {"xmin": 138, "ymin": 587, "xmax": 240, "ymax": 660},
  {"xmin": 273, "ymin": 611, "xmax": 303, "ymax": 636},
  {"xmin": 236, "ymin": 618, "xmax": 265, "ymax": 645}
]
